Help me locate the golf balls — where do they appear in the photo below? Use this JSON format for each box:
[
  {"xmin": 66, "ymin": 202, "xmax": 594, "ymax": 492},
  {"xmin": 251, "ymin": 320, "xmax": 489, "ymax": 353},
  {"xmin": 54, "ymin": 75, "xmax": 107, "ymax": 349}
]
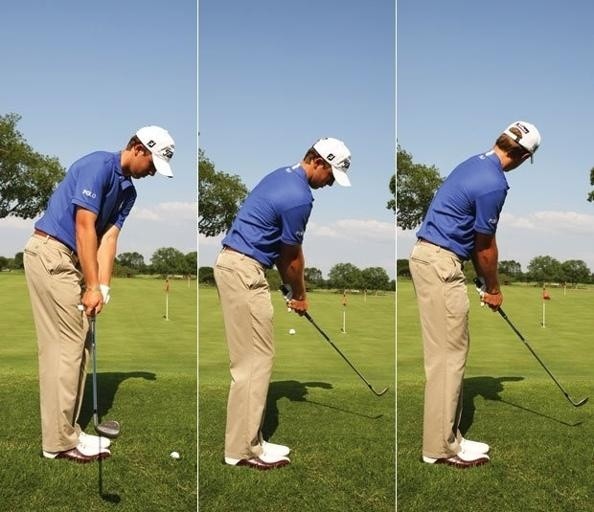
[
  {"xmin": 170, "ymin": 451, "xmax": 179, "ymax": 458},
  {"xmin": 289, "ymin": 328, "xmax": 294, "ymax": 334}
]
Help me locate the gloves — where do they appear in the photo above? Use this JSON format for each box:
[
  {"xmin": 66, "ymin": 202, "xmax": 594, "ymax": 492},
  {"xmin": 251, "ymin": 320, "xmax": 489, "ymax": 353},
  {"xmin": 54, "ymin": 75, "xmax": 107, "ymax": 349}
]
[
  {"xmin": 78, "ymin": 284, "xmax": 110, "ymax": 310},
  {"xmin": 474, "ymin": 277, "xmax": 487, "ymax": 307},
  {"xmin": 280, "ymin": 284, "xmax": 294, "ymax": 313}
]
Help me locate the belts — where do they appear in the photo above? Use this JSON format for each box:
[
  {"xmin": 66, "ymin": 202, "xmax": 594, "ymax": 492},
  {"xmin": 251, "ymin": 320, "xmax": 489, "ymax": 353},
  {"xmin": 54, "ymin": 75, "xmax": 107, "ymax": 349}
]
[{"xmin": 35, "ymin": 230, "xmax": 55, "ymax": 240}]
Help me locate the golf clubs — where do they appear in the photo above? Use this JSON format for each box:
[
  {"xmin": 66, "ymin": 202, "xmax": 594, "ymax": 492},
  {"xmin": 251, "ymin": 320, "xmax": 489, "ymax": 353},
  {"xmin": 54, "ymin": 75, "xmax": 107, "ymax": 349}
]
[
  {"xmin": 279, "ymin": 284, "xmax": 388, "ymax": 396},
  {"xmin": 91, "ymin": 308, "xmax": 119, "ymax": 438},
  {"xmin": 473, "ymin": 276, "xmax": 588, "ymax": 407}
]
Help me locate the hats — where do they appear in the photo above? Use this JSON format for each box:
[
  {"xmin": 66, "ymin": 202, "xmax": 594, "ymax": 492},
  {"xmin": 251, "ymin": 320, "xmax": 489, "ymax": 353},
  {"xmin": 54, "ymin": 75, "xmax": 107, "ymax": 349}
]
[
  {"xmin": 136, "ymin": 126, "xmax": 176, "ymax": 177},
  {"xmin": 503, "ymin": 121, "xmax": 541, "ymax": 163},
  {"xmin": 313, "ymin": 138, "xmax": 351, "ymax": 188}
]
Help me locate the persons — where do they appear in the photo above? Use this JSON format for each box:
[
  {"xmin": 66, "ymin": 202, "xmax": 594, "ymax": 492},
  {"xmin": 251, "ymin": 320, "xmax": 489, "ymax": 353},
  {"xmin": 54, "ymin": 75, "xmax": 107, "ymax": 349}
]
[
  {"xmin": 409, "ymin": 120, "xmax": 540, "ymax": 467},
  {"xmin": 21, "ymin": 126, "xmax": 175, "ymax": 464},
  {"xmin": 212, "ymin": 139, "xmax": 350, "ymax": 470}
]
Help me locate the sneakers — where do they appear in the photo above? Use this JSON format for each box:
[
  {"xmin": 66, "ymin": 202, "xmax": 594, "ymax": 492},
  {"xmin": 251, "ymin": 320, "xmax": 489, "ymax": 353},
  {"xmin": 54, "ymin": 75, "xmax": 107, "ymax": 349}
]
[
  {"xmin": 42, "ymin": 432, "xmax": 110, "ymax": 461},
  {"xmin": 422, "ymin": 438, "xmax": 490, "ymax": 468},
  {"xmin": 224, "ymin": 441, "xmax": 290, "ymax": 469}
]
